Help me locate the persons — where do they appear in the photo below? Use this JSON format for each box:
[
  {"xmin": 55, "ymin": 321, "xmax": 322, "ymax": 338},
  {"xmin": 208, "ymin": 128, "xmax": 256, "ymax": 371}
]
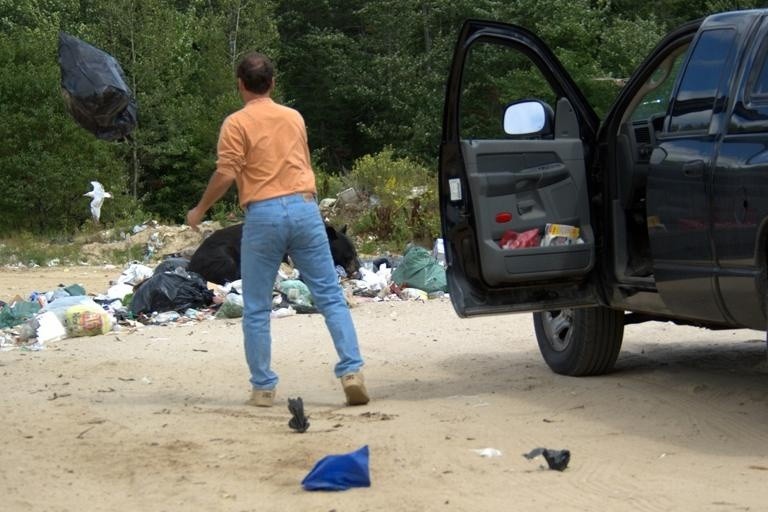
[{"xmin": 187, "ymin": 53, "xmax": 369, "ymax": 406}]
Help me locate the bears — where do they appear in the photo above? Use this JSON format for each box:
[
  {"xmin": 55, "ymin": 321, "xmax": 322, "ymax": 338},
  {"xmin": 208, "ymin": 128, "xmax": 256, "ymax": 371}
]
[{"xmin": 186, "ymin": 221, "xmax": 364, "ymax": 286}]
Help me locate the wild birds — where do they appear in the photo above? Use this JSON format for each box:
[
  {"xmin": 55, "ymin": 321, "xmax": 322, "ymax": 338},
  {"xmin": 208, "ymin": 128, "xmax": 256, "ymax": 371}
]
[{"xmin": 82, "ymin": 181, "xmax": 114, "ymax": 223}]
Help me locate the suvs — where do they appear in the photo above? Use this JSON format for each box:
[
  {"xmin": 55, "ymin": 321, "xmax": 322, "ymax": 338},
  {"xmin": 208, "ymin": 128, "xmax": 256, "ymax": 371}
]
[{"xmin": 434, "ymin": 7, "xmax": 766, "ymax": 380}]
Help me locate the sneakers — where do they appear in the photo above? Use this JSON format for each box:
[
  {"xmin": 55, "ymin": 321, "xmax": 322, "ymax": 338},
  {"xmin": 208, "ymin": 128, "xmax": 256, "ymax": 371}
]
[
  {"xmin": 341, "ymin": 373, "xmax": 369, "ymax": 405},
  {"xmin": 251, "ymin": 390, "xmax": 274, "ymax": 407}
]
